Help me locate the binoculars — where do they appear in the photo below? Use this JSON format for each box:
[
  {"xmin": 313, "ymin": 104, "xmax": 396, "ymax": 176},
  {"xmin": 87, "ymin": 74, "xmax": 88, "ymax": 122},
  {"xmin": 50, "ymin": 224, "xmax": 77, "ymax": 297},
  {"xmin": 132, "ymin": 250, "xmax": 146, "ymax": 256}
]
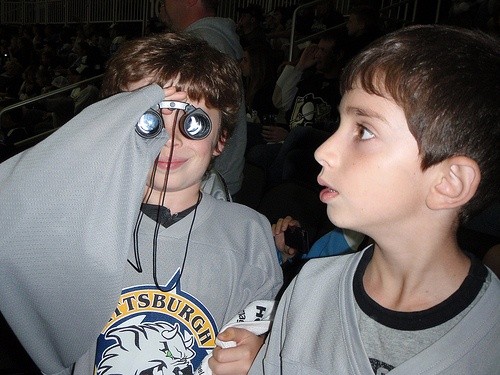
[{"xmin": 135, "ymin": 99, "xmax": 213, "ymax": 141}]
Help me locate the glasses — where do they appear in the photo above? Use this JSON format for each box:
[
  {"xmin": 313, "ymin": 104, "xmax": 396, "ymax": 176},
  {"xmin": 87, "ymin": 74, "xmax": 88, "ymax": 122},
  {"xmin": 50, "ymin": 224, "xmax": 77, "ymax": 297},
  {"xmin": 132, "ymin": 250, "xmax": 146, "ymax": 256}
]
[{"xmin": 312, "ymin": 47, "xmax": 334, "ymax": 55}]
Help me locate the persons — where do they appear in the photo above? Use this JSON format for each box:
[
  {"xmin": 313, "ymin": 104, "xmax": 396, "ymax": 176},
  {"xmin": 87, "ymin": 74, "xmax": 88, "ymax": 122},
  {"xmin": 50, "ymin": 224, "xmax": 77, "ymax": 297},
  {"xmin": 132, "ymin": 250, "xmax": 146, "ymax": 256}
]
[
  {"xmin": 0, "ymin": 17, "xmax": 176, "ymax": 158},
  {"xmin": 159, "ymin": 0, "xmax": 252, "ymax": 206},
  {"xmin": 1, "ymin": 31, "xmax": 281, "ymax": 375},
  {"xmin": 440, "ymin": 0, "xmax": 491, "ymax": 35},
  {"xmin": 250, "ymin": 22, "xmax": 500, "ymax": 375},
  {"xmin": 232, "ymin": 0, "xmax": 393, "ymax": 306}
]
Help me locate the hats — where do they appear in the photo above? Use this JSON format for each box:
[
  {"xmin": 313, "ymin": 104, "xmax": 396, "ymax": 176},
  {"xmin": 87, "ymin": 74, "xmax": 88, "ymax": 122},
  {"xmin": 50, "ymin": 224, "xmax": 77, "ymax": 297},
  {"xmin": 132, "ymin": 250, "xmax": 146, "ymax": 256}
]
[{"xmin": 236, "ymin": 4, "xmax": 266, "ymax": 23}]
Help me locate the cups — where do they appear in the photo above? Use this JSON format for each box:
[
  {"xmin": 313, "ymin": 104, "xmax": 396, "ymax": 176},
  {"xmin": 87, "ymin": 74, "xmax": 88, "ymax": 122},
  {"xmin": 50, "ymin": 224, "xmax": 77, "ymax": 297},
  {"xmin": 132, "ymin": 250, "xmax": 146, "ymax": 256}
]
[{"xmin": 263, "ymin": 113, "xmax": 278, "ymax": 127}]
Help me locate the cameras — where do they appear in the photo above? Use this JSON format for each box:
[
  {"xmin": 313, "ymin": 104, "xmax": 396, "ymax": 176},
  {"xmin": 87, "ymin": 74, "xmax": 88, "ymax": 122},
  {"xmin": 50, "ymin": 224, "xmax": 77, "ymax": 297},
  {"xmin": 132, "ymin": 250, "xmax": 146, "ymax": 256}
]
[{"xmin": 284, "ymin": 225, "xmax": 310, "ymax": 254}]
